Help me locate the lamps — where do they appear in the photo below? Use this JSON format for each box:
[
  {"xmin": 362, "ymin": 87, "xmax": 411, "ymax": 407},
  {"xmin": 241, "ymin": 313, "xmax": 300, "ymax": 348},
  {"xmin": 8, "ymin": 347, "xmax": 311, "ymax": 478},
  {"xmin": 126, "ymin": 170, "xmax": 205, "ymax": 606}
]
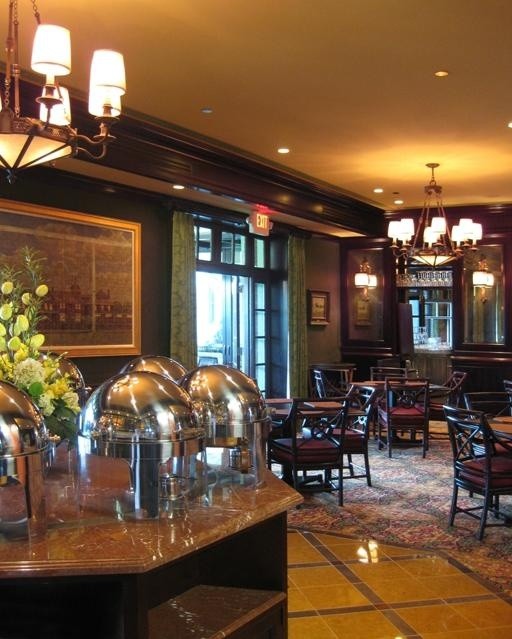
[
  {"xmin": 472, "ymin": 255, "xmax": 486, "ymax": 303},
  {"xmin": 387, "ymin": 163, "xmax": 483, "ymax": 270},
  {"xmin": 0, "ymin": 0, "xmax": 127, "ymax": 185},
  {"xmin": 485, "ymin": 261, "xmax": 495, "ymax": 302},
  {"xmin": 368, "ymin": 265, "xmax": 379, "ymax": 290},
  {"xmin": 354, "ymin": 256, "xmax": 369, "ymax": 302}
]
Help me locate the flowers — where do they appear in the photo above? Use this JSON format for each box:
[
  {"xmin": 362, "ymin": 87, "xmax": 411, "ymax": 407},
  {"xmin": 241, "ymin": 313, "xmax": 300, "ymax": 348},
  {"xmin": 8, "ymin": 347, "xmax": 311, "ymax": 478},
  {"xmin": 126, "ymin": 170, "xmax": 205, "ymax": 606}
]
[{"xmin": 0, "ymin": 244, "xmax": 88, "ymax": 443}]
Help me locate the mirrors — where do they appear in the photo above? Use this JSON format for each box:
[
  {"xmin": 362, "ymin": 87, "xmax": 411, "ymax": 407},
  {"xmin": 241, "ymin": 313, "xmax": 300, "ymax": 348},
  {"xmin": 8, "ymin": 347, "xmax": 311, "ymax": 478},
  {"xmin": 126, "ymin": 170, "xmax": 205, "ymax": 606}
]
[
  {"xmin": 339, "ymin": 238, "xmax": 397, "ymax": 357},
  {"xmin": 451, "ymin": 229, "xmax": 512, "ymax": 350}
]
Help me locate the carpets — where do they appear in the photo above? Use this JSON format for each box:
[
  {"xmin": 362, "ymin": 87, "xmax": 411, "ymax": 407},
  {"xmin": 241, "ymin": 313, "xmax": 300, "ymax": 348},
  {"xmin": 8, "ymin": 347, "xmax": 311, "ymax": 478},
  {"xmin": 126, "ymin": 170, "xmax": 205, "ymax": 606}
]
[{"xmin": 266, "ymin": 431, "xmax": 512, "ymax": 606}]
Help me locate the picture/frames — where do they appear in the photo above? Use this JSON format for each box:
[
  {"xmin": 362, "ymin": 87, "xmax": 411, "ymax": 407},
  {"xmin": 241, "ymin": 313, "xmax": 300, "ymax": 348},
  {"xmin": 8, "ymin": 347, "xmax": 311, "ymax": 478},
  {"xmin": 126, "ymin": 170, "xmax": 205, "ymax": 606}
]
[
  {"xmin": 306, "ymin": 287, "xmax": 331, "ymax": 327},
  {"xmin": 0, "ymin": 198, "xmax": 143, "ymax": 358},
  {"xmin": 353, "ymin": 297, "xmax": 373, "ymax": 327}
]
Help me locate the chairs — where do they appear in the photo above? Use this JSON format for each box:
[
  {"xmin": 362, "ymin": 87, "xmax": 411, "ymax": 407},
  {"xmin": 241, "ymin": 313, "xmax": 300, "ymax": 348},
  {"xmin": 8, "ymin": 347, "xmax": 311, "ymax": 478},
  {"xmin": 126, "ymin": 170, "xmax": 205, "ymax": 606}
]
[
  {"xmin": 503, "ymin": 378, "xmax": 512, "ymax": 394},
  {"xmin": 320, "ymin": 383, "xmax": 379, "ymax": 488},
  {"xmin": 463, "ymin": 389, "xmax": 512, "ymax": 512},
  {"xmin": 441, "ymin": 404, "xmax": 512, "ymax": 540},
  {"xmin": 412, "ymin": 370, "xmax": 469, "ymax": 438},
  {"xmin": 265, "ymin": 395, "xmax": 354, "ymax": 509},
  {"xmin": 376, "ymin": 376, "xmax": 431, "ymax": 459},
  {"xmin": 369, "ymin": 366, "xmax": 422, "ymax": 380},
  {"xmin": 312, "ymin": 368, "xmax": 372, "ymax": 442}
]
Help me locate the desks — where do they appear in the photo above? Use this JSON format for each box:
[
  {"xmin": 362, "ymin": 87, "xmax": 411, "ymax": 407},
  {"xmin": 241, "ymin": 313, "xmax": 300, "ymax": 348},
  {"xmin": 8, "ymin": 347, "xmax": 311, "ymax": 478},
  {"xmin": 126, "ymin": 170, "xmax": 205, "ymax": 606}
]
[
  {"xmin": 347, "ymin": 381, "xmax": 452, "ymax": 448},
  {"xmin": 260, "ymin": 397, "xmax": 369, "ymax": 492},
  {"xmin": 460, "ymin": 416, "xmax": 512, "ymax": 521}
]
